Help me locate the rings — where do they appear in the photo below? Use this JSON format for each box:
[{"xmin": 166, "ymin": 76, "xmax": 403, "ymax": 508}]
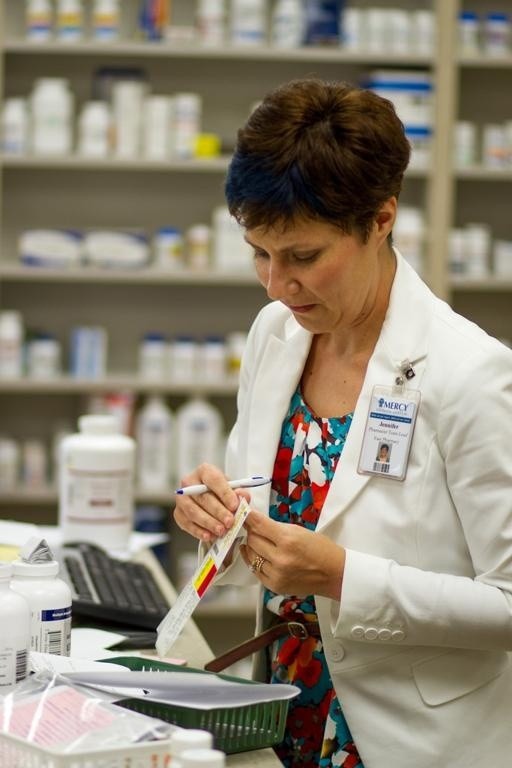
[
  {"xmin": 248, "ymin": 565, "xmax": 257, "ymax": 572},
  {"xmin": 252, "ymin": 557, "xmax": 266, "ymax": 572}
]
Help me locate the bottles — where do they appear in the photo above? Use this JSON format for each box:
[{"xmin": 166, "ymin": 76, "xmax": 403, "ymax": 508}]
[
  {"xmin": 1, "ymin": 1, "xmax": 511, "ymax": 504},
  {"xmin": 55, "ymin": 412, "xmax": 138, "ymax": 563},
  {"xmin": 0, "ymin": 559, "xmax": 74, "ymax": 699},
  {"xmin": 165, "ymin": 728, "xmax": 227, "ymax": 768}
]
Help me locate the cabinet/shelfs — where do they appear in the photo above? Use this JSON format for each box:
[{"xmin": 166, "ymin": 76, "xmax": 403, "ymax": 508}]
[{"xmin": 2, "ymin": 1, "xmax": 510, "ymax": 680}]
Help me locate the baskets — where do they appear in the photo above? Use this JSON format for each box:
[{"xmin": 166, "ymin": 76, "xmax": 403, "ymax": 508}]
[
  {"xmin": 0, "ymin": 715, "xmax": 181, "ymax": 768},
  {"xmin": 73, "ymin": 657, "xmax": 290, "ymax": 755}
]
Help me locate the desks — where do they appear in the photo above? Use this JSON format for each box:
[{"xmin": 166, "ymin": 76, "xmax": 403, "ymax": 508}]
[{"xmin": 4, "ymin": 548, "xmax": 288, "ymax": 766}]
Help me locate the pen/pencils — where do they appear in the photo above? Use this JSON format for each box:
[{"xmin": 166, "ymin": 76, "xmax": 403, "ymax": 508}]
[{"xmin": 174, "ymin": 476, "xmax": 274, "ymax": 496}]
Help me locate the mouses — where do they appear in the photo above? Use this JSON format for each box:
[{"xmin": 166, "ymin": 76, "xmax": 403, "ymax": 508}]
[{"xmin": 65, "ymin": 541, "xmax": 106, "ymax": 556}]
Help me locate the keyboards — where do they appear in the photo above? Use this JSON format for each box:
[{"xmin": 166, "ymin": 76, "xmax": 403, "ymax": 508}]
[{"xmin": 54, "ymin": 546, "xmax": 170, "ymax": 629}]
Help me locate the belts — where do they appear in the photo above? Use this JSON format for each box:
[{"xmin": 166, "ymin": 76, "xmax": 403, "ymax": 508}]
[{"xmin": 206, "ymin": 620, "xmax": 320, "ymax": 674}]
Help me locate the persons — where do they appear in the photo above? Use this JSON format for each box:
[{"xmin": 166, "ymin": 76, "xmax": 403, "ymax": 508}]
[
  {"xmin": 166, "ymin": 78, "xmax": 512, "ymax": 768},
  {"xmin": 377, "ymin": 444, "xmax": 390, "ymax": 464}
]
